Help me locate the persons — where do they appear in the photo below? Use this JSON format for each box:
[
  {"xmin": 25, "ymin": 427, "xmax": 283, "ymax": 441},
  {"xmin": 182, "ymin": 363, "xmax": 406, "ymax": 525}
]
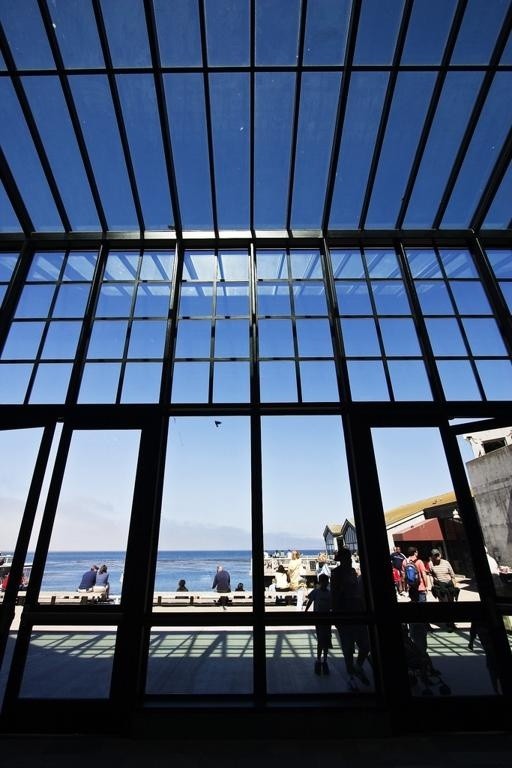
[
  {"xmin": 93, "ymin": 563, "xmax": 114, "ymax": 602},
  {"xmin": 174, "ymin": 579, "xmax": 189, "ymax": 598},
  {"xmin": 211, "ymin": 565, "xmax": 231, "ymax": 593},
  {"xmin": 287, "ymin": 551, "xmax": 307, "ymax": 591},
  {"xmin": 2, "ymin": 572, "xmax": 10, "ymax": 592},
  {"xmin": 280, "ymin": 550, "xmax": 285, "ymax": 556},
  {"xmin": 274, "ymin": 550, "xmax": 280, "ymax": 558},
  {"xmin": 286, "ymin": 549, "xmax": 291, "ymax": 558},
  {"xmin": 301, "ymin": 544, "xmax": 512, "ymax": 691},
  {"xmin": 274, "ymin": 565, "xmax": 290, "ymax": 592},
  {"xmin": 234, "ymin": 583, "xmax": 245, "ymax": 598},
  {"xmin": 77, "ymin": 565, "xmax": 99, "ymax": 593}
]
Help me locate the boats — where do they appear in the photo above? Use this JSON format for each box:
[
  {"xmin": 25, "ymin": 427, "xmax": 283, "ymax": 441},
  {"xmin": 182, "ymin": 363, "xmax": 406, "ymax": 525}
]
[{"xmin": 0, "ymin": 554, "xmax": 33, "ymax": 584}]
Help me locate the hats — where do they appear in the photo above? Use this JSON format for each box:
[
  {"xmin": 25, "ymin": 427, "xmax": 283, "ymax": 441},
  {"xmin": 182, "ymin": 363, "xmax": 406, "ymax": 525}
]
[{"xmin": 432, "ymin": 549, "xmax": 440, "ymax": 556}]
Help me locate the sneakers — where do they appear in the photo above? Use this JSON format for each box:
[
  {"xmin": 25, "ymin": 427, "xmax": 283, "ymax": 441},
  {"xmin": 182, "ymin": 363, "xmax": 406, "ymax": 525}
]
[{"xmin": 314, "ymin": 661, "xmax": 370, "ymax": 686}]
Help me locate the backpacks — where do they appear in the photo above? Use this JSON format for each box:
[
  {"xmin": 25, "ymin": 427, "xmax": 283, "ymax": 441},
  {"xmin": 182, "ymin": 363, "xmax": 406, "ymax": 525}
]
[{"xmin": 405, "ymin": 559, "xmax": 420, "ymax": 586}]
